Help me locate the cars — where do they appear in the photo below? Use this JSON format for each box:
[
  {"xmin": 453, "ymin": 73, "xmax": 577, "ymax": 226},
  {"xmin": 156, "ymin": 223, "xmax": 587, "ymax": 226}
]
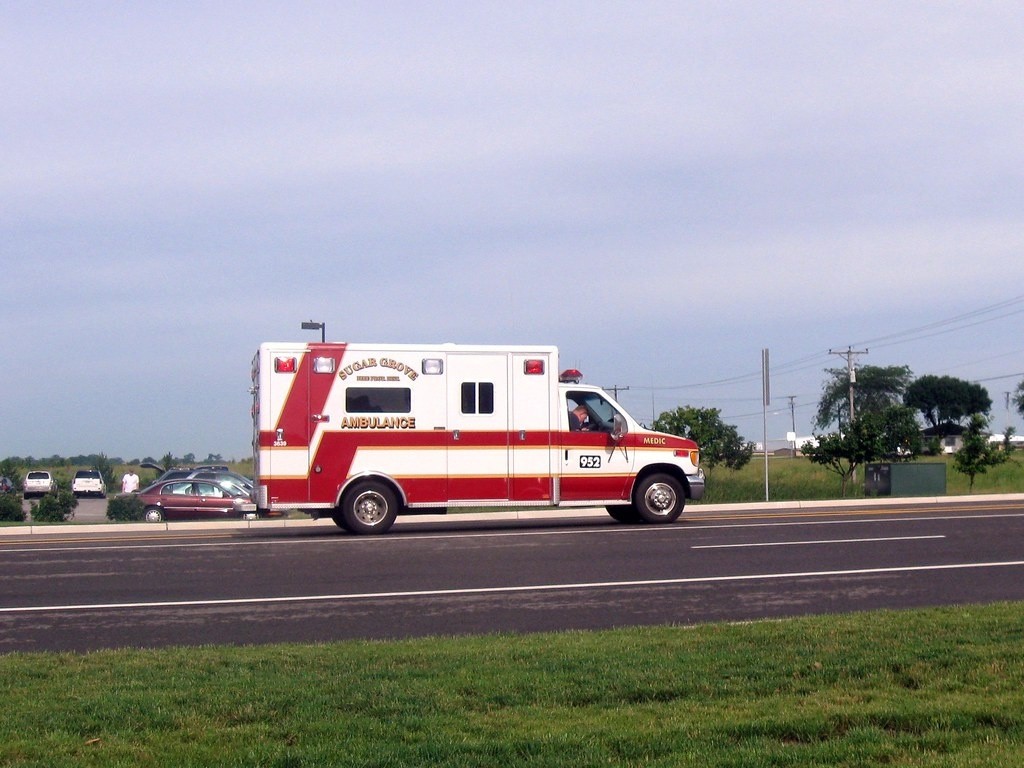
[
  {"xmin": 141, "ymin": 462, "xmax": 255, "ymax": 499},
  {"xmin": 113, "ymin": 478, "xmax": 259, "ymax": 524},
  {"xmin": 0, "ymin": 476, "xmax": 16, "ymax": 497}
]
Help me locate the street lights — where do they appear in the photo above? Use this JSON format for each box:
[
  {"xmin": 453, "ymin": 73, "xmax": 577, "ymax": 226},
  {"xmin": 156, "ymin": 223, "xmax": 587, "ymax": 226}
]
[{"xmin": 301, "ymin": 322, "xmax": 326, "ymax": 344}]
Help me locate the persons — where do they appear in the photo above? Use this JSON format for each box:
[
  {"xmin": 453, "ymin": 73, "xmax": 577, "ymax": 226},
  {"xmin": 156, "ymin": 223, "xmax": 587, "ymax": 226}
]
[
  {"xmin": 568, "ymin": 405, "xmax": 589, "ymax": 432},
  {"xmin": 121, "ymin": 468, "xmax": 140, "ymax": 493}
]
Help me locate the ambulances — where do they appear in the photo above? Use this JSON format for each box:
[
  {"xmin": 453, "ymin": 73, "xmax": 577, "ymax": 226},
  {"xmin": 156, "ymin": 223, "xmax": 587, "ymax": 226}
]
[{"xmin": 247, "ymin": 342, "xmax": 708, "ymax": 537}]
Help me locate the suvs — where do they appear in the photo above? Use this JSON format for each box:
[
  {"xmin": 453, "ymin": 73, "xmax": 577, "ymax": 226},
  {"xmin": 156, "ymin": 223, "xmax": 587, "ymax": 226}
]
[
  {"xmin": 71, "ymin": 468, "xmax": 107, "ymax": 498},
  {"xmin": 23, "ymin": 469, "xmax": 59, "ymax": 499}
]
